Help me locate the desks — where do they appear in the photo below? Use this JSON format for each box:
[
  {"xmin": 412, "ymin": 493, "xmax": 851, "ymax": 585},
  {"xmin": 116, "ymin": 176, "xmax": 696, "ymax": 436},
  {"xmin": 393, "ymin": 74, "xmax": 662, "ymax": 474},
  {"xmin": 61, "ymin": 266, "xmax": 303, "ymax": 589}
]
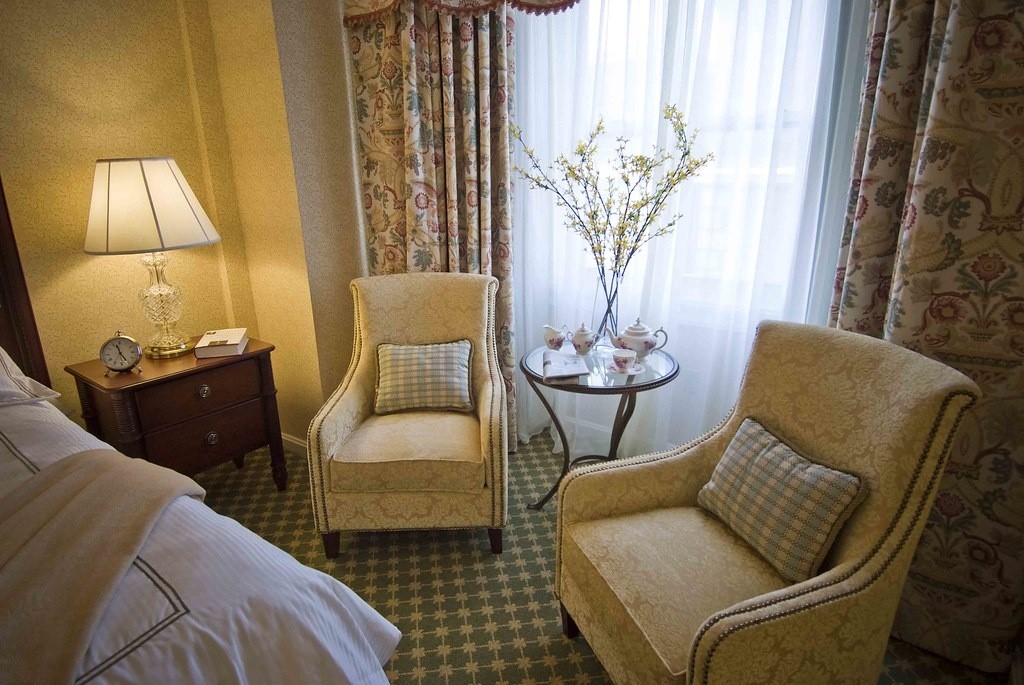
[{"xmin": 520, "ymin": 333, "xmax": 681, "ymax": 510}]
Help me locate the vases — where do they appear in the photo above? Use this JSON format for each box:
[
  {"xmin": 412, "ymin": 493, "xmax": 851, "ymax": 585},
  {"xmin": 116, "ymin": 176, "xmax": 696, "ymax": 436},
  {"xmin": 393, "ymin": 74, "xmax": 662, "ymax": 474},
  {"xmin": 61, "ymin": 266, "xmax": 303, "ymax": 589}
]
[{"xmin": 590, "ymin": 275, "xmax": 623, "ymax": 357}]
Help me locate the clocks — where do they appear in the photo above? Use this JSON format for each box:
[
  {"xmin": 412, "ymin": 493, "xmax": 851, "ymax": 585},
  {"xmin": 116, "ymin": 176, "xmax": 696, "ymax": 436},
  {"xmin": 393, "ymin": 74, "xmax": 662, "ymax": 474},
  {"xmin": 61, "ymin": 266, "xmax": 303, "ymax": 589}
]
[{"xmin": 99, "ymin": 331, "xmax": 147, "ymax": 378}]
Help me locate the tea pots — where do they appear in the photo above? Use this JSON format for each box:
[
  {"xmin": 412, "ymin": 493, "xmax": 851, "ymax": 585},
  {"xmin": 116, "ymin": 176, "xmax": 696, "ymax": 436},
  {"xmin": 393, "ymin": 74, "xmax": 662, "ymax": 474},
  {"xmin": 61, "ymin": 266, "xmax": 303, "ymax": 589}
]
[
  {"xmin": 566, "ymin": 323, "xmax": 603, "ymax": 355},
  {"xmin": 604, "ymin": 318, "xmax": 668, "ymax": 358}
]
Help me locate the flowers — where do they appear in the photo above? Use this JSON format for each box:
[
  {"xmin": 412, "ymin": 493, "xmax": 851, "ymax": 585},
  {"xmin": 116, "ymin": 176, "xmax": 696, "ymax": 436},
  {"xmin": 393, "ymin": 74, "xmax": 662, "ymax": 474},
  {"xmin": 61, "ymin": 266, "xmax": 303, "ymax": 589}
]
[{"xmin": 507, "ymin": 98, "xmax": 716, "ymax": 339}]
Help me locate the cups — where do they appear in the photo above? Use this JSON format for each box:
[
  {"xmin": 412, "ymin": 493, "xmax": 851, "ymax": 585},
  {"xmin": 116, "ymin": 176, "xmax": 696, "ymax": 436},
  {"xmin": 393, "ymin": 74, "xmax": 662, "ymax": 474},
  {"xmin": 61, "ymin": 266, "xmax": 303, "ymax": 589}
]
[{"xmin": 613, "ymin": 349, "xmax": 637, "ymax": 373}]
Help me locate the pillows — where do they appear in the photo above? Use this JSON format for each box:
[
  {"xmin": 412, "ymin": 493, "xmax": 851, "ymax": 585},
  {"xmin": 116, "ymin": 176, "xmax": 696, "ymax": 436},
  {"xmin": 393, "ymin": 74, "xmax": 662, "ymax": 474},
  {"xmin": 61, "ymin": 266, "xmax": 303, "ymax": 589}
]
[
  {"xmin": 371, "ymin": 337, "xmax": 477, "ymax": 415},
  {"xmin": 698, "ymin": 414, "xmax": 870, "ymax": 585},
  {"xmin": 0, "ymin": 344, "xmax": 62, "ymax": 407}
]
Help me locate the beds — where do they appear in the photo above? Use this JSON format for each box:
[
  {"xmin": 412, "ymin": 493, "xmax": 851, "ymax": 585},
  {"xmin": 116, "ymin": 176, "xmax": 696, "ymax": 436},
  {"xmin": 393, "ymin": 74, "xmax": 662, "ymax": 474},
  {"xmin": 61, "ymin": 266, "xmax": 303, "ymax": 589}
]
[{"xmin": 0, "ymin": 345, "xmax": 403, "ymax": 685}]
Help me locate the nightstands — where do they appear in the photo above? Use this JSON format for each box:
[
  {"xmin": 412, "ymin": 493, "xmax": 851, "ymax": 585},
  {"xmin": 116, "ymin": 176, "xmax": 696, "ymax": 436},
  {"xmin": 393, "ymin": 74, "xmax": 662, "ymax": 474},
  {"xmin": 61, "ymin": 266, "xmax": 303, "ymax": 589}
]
[{"xmin": 59, "ymin": 330, "xmax": 291, "ymax": 491}]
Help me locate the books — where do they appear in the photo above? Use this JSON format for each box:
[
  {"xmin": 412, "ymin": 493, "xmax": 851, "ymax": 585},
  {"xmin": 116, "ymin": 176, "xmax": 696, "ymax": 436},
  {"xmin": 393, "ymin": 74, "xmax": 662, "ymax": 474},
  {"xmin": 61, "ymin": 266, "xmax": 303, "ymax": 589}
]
[{"xmin": 194, "ymin": 328, "xmax": 250, "ymax": 360}]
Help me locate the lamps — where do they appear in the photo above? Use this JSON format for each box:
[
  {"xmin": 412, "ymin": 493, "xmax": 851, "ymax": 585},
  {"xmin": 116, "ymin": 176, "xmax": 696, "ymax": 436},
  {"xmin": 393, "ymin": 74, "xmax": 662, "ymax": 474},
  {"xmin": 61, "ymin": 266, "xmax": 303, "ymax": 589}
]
[{"xmin": 82, "ymin": 158, "xmax": 223, "ymax": 360}]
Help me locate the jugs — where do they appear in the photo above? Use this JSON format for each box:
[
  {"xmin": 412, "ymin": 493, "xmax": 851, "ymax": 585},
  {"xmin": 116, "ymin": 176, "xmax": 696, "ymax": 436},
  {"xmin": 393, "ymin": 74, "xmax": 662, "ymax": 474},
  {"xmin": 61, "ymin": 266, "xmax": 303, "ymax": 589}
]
[{"xmin": 542, "ymin": 323, "xmax": 568, "ymax": 351}]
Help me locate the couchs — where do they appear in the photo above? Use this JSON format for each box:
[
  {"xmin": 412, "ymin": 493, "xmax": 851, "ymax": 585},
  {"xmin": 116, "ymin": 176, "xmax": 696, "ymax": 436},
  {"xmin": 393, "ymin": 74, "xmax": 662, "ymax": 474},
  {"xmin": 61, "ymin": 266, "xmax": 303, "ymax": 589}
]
[
  {"xmin": 303, "ymin": 271, "xmax": 509, "ymax": 558},
  {"xmin": 555, "ymin": 320, "xmax": 984, "ymax": 685}
]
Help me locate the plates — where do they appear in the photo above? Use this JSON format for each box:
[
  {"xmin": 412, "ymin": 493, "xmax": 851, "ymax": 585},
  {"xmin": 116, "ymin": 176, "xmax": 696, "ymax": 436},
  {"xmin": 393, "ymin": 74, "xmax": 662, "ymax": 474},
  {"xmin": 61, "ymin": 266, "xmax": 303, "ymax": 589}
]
[{"xmin": 606, "ymin": 362, "xmax": 646, "ymax": 375}]
